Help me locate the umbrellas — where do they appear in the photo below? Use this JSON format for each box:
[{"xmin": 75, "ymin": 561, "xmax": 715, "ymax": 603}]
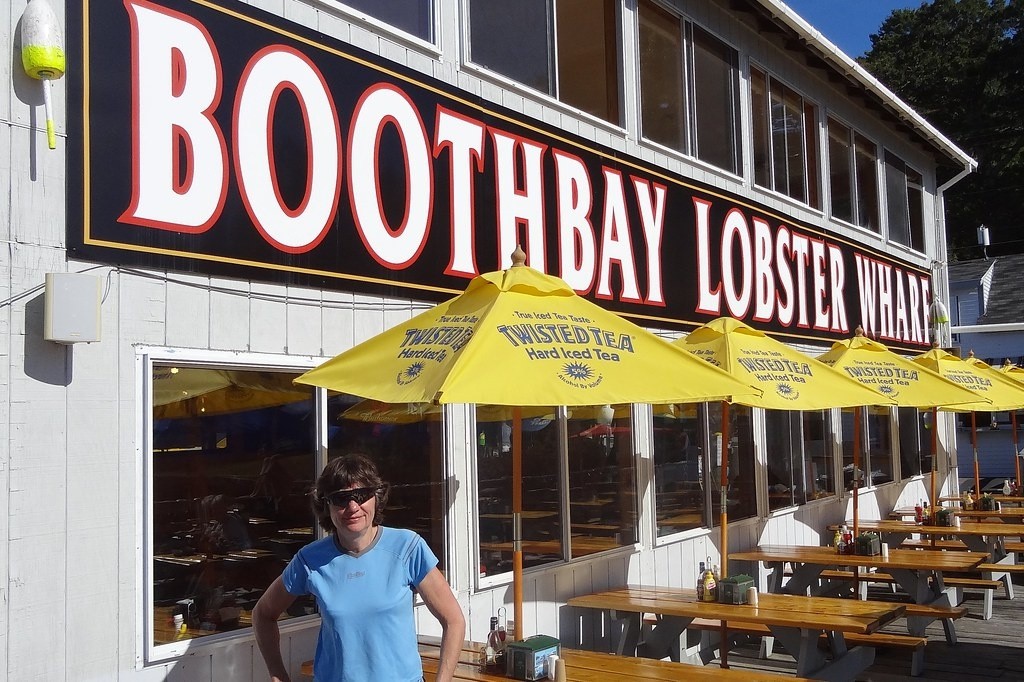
[
  {"xmin": 672, "ymin": 315, "xmax": 1023, "ymax": 670},
  {"xmin": 153, "ymin": 366, "xmax": 701, "ymax": 424},
  {"xmin": 293, "ymin": 242, "xmax": 766, "ymax": 643}
]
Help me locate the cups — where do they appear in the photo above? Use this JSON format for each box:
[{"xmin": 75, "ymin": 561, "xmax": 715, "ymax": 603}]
[{"xmin": 746, "ymin": 588, "xmax": 758, "ymax": 604}]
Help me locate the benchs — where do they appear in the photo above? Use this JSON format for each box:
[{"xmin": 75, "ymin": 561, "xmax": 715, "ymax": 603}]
[
  {"xmin": 900, "ymin": 540, "xmax": 1024, "ymax": 553},
  {"xmin": 896, "ymin": 603, "xmax": 969, "ymax": 619},
  {"xmin": 783, "ymin": 570, "xmax": 1005, "ymax": 600},
  {"xmin": 976, "ymin": 563, "xmax": 1024, "ymax": 574},
  {"xmin": 642, "ymin": 615, "xmax": 928, "ymax": 652}
]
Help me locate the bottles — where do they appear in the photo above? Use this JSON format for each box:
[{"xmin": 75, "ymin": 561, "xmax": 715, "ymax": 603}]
[
  {"xmin": 965, "ymin": 496, "xmax": 974, "ymax": 511},
  {"xmin": 703, "ymin": 557, "xmax": 712, "ymax": 601},
  {"xmin": 704, "ymin": 571, "xmax": 716, "ymax": 601},
  {"xmin": 714, "ymin": 565, "xmax": 719, "ymax": 601},
  {"xmin": 499, "ymin": 626, "xmax": 506, "ymax": 642},
  {"xmin": 505, "ymin": 621, "xmax": 514, "ymax": 648},
  {"xmin": 697, "ymin": 562, "xmax": 705, "ymax": 601},
  {"xmin": 1003, "ymin": 480, "xmax": 1010, "ymax": 495},
  {"xmin": 487, "ymin": 617, "xmax": 502, "ymax": 665},
  {"xmin": 923, "ymin": 510, "xmax": 928, "ymax": 517},
  {"xmin": 834, "ymin": 525, "xmax": 854, "ymax": 554}
]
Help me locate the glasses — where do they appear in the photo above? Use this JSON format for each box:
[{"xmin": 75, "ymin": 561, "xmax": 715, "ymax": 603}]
[{"xmin": 322, "ymin": 486, "xmax": 375, "ymax": 508}]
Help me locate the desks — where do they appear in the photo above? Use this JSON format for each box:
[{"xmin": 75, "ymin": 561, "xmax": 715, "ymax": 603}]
[
  {"xmin": 152, "ymin": 478, "xmax": 834, "ymax": 645},
  {"xmin": 303, "ymin": 486, "xmax": 1024, "ymax": 682}
]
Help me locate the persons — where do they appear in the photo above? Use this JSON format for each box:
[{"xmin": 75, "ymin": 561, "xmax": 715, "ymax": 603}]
[{"xmin": 251, "ymin": 453, "xmax": 467, "ymax": 682}]
